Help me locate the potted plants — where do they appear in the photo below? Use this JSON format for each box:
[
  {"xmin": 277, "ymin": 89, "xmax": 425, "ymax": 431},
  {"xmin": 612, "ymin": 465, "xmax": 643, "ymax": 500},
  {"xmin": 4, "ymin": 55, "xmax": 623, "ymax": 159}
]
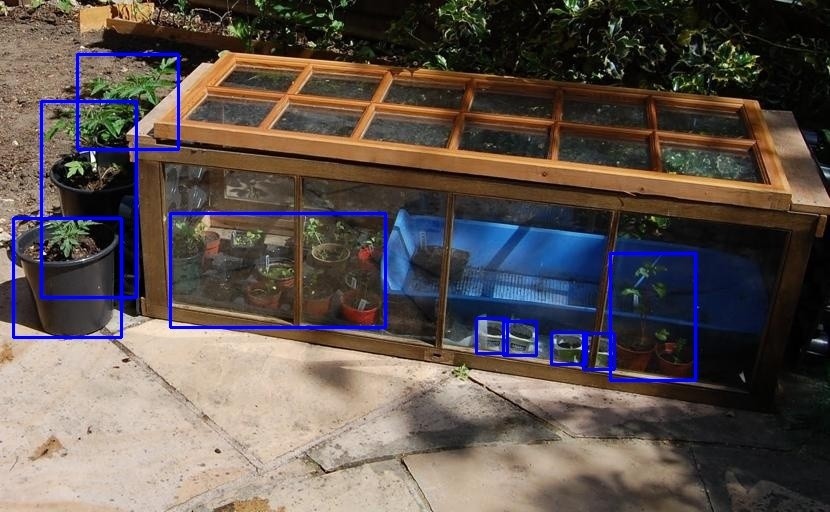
[
  {"xmin": 611, "ymin": 254, "xmax": 695, "ymax": 381},
  {"xmin": 171, "ymin": 214, "xmax": 385, "ymax": 328},
  {"xmin": 41, "ymin": 102, "xmax": 137, "ymax": 297},
  {"xmin": 78, "ymin": 54, "xmax": 178, "ymax": 149},
  {"xmin": 14, "ymin": 219, "xmax": 122, "ymax": 337}
]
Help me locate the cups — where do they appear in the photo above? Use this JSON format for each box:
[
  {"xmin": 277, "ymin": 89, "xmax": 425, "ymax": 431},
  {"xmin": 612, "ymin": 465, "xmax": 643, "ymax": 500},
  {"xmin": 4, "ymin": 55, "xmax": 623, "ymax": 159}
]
[
  {"xmin": 583, "ymin": 334, "xmax": 615, "ymax": 369},
  {"xmin": 503, "ymin": 321, "xmax": 536, "ymax": 356},
  {"xmin": 476, "ymin": 319, "xmax": 508, "ymax": 353},
  {"xmin": 552, "ymin": 333, "xmax": 586, "ymax": 365}
]
